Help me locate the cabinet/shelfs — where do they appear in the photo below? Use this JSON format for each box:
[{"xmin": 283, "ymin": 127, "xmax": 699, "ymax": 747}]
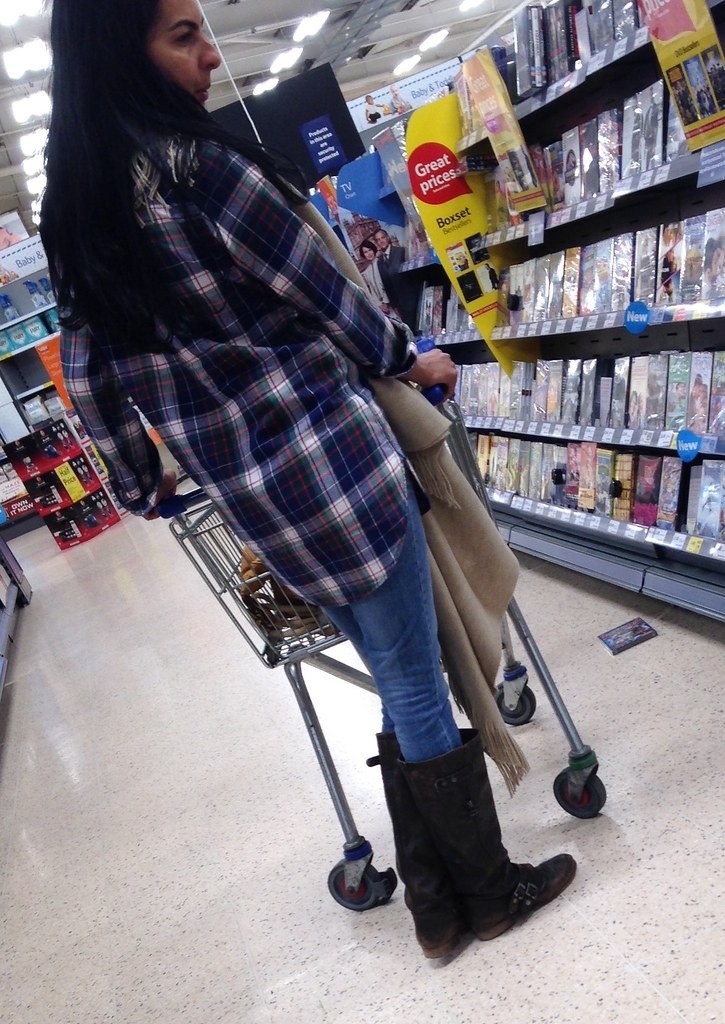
[
  {"xmin": 0, "ymin": 206, "xmax": 93, "ymax": 527},
  {"xmin": 586, "ymin": 0, "xmax": 725, "ymax": 622},
  {"xmin": 480, "ymin": 62, "xmax": 660, "ymax": 620},
  {"xmin": 365, "ymin": 122, "xmax": 513, "ymax": 548}
]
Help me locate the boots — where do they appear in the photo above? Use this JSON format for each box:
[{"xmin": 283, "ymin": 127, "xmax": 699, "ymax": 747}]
[{"xmin": 375, "ymin": 730, "xmax": 577, "ymax": 959}]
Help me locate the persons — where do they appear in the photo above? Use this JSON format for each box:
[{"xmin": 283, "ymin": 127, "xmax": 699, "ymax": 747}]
[
  {"xmin": 357, "ymin": 229, "xmax": 422, "ymax": 332},
  {"xmin": 362, "ymin": 86, "xmax": 413, "ymax": 124},
  {"xmin": 432, "ymin": 85, "xmax": 725, "ymax": 537},
  {"xmin": 44, "ymin": 0, "xmax": 576, "ymax": 971}
]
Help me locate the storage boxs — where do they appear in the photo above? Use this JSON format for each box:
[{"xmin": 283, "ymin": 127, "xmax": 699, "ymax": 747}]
[
  {"xmin": 1, "ymin": 309, "xmax": 121, "ymax": 552},
  {"xmin": 398, "ymin": 0, "xmax": 725, "ymax": 542}
]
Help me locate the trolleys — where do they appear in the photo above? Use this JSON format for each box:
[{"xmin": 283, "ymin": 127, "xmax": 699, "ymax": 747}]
[{"xmin": 155, "ymin": 337, "xmax": 608, "ymax": 912}]
